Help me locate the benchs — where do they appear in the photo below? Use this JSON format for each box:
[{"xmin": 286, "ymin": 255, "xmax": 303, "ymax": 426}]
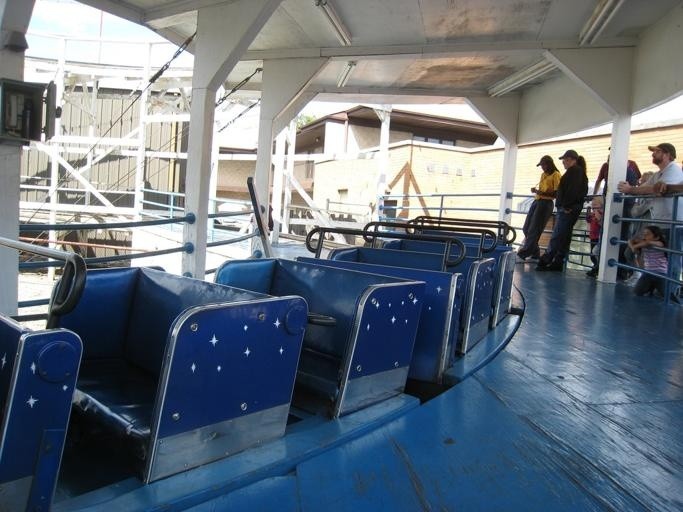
[
  {"xmin": 297, "ymin": 213, "xmax": 517, "ymax": 386},
  {"xmin": 3, "ymin": 316, "xmax": 83, "ymax": 509},
  {"xmin": 214, "ymin": 258, "xmax": 426, "ymax": 420},
  {"xmin": 44, "ymin": 267, "xmax": 308, "ymax": 483}
]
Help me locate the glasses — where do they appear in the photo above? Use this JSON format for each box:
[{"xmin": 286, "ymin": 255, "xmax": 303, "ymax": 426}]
[
  {"xmin": 335, "ymin": 60, "xmax": 358, "ymax": 91},
  {"xmin": 576, "ymin": 1, "xmax": 626, "ymax": 51},
  {"xmin": 313, "ymin": 1, "xmax": 355, "ymax": 48},
  {"xmin": 486, "ymin": 52, "xmax": 561, "ymax": 99}
]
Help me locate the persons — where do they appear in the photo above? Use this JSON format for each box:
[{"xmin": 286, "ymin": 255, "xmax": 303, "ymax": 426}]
[
  {"xmin": 586, "ymin": 142, "xmax": 683, "ymax": 305},
  {"xmin": 535, "ymin": 150, "xmax": 588, "ymax": 272},
  {"xmin": 516, "ymin": 155, "xmax": 562, "ymax": 260}
]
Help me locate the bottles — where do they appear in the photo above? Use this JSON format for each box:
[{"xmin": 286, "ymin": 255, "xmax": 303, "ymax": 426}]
[
  {"xmin": 537, "ymin": 155, "xmax": 553, "ymax": 166},
  {"xmin": 648, "ymin": 143, "xmax": 676, "ymax": 158},
  {"xmin": 558, "ymin": 150, "xmax": 578, "ymax": 159}
]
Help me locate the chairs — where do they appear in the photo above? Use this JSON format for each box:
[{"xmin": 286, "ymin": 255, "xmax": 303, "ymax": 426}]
[{"xmin": 535, "ymin": 256, "xmax": 563, "ymax": 272}]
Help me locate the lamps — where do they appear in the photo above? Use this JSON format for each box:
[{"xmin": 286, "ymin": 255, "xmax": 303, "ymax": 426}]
[
  {"xmin": 313, "ymin": 1, "xmax": 355, "ymax": 48},
  {"xmin": 335, "ymin": 60, "xmax": 358, "ymax": 91},
  {"xmin": 486, "ymin": 52, "xmax": 561, "ymax": 99},
  {"xmin": 576, "ymin": 1, "xmax": 626, "ymax": 51}
]
